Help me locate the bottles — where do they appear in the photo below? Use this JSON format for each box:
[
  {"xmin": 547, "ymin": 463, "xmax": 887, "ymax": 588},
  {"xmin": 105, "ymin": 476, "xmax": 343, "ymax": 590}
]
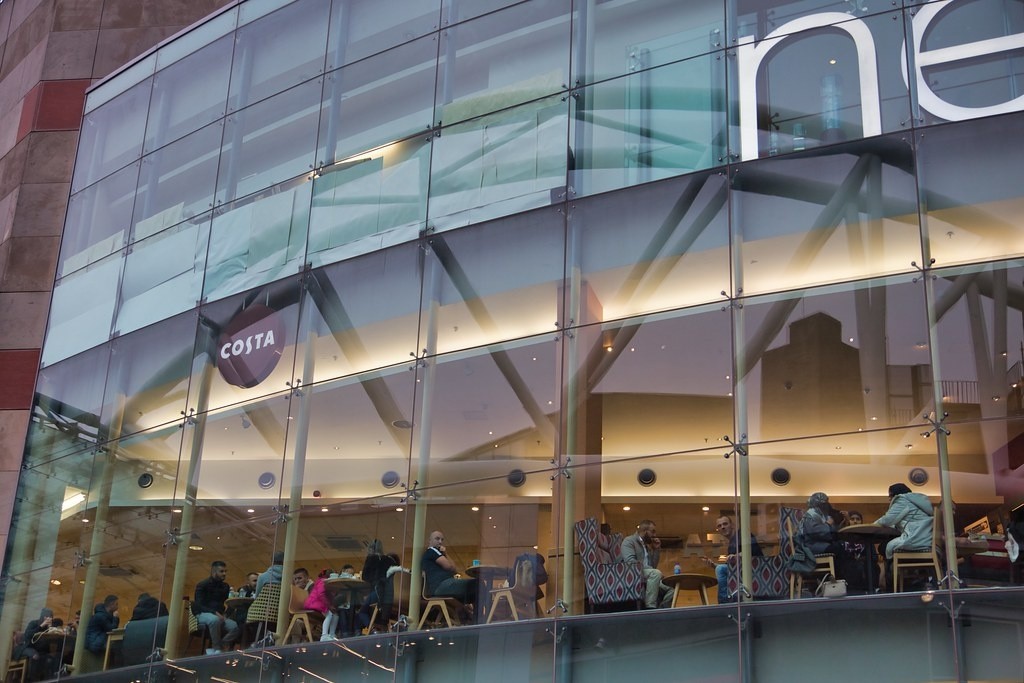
[
  {"xmin": 228, "ymin": 587, "xmax": 235, "ymax": 598},
  {"xmin": 674, "ymin": 562, "xmax": 680, "ymax": 575},
  {"xmin": 239, "ymin": 587, "xmax": 245, "ymax": 598}
]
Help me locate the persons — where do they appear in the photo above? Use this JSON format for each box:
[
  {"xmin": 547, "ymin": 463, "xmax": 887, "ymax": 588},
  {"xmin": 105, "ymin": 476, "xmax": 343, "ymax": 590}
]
[
  {"xmin": 193, "ymin": 561, "xmax": 262, "ymax": 655},
  {"xmin": 250, "ymin": 552, "xmax": 315, "ymax": 648},
  {"xmin": 615, "ymin": 520, "xmax": 675, "ymax": 610},
  {"xmin": 701, "ymin": 515, "xmax": 763, "ymax": 604},
  {"xmin": 15, "ymin": 608, "xmax": 82, "ymax": 681},
  {"xmin": 786, "ymin": 492, "xmax": 881, "ymax": 593},
  {"xmin": 86, "ymin": 596, "xmax": 122, "ymax": 669},
  {"xmin": 131, "ymin": 593, "xmax": 170, "ymax": 621},
  {"xmin": 304, "ymin": 565, "xmax": 362, "ymax": 642},
  {"xmin": 874, "ymin": 483, "xmax": 968, "ymax": 592},
  {"xmin": 359, "ymin": 539, "xmax": 400, "ymax": 634},
  {"xmin": 422, "ymin": 531, "xmax": 485, "ymax": 624}
]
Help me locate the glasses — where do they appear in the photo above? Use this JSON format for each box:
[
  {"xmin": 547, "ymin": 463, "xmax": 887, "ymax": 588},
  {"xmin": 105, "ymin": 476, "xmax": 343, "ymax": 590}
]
[
  {"xmin": 294, "ymin": 576, "xmax": 305, "ymax": 581},
  {"xmin": 647, "ymin": 528, "xmax": 656, "ymax": 534}
]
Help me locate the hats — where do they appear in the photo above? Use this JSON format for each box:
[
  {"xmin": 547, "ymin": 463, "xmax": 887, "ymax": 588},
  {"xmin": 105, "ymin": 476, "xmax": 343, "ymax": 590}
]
[{"xmin": 41, "ymin": 608, "xmax": 53, "ymax": 617}]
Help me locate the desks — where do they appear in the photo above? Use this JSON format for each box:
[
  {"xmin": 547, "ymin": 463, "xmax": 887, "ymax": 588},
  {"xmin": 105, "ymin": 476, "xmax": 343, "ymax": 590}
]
[
  {"xmin": 661, "ymin": 573, "xmax": 719, "ymax": 608},
  {"xmin": 102, "ymin": 630, "xmax": 125, "ymax": 671},
  {"xmin": 225, "ymin": 597, "xmax": 255, "ymax": 649},
  {"xmin": 838, "ymin": 523, "xmax": 900, "ymax": 595},
  {"xmin": 465, "ymin": 564, "xmax": 512, "ymax": 624},
  {"xmin": 36, "ymin": 633, "xmax": 75, "ymax": 677},
  {"xmin": 955, "ymin": 541, "xmax": 990, "ymax": 587},
  {"xmin": 324, "ymin": 578, "xmax": 371, "ymax": 637}
]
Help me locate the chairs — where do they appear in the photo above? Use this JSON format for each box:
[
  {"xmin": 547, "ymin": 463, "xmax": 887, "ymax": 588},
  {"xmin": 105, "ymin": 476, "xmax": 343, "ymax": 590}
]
[
  {"xmin": 246, "ymin": 582, "xmax": 281, "ymax": 647},
  {"xmin": 182, "ymin": 600, "xmax": 225, "ymax": 657},
  {"xmin": 1, "ymin": 631, "xmax": 29, "ymax": 683},
  {"xmin": 417, "ymin": 570, "xmax": 463, "ymax": 630},
  {"xmin": 893, "ymin": 505, "xmax": 947, "ymax": 593},
  {"xmin": 281, "ymin": 583, "xmax": 325, "ymax": 645},
  {"xmin": 787, "ymin": 515, "xmax": 835, "ymax": 600},
  {"xmin": 484, "ymin": 559, "xmax": 537, "ymax": 624},
  {"xmin": 367, "ymin": 571, "xmax": 411, "ymax": 635},
  {"xmin": 123, "ymin": 614, "xmax": 168, "ymax": 664}
]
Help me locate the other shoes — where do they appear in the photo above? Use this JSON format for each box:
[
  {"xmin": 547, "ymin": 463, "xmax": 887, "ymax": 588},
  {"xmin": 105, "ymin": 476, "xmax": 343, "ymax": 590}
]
[
  {"xmin": 474, "ymin": 615, "xmax": 486, "ymax": 623},
  {"xmin": 319, "ymin": 634, "xmax": 339, "ymax": 642},
  {"xmin": 214, "ymin": 650, "xmax": 221, "ymax": 654},
  {"xmin": 221, "ymin": 644, "xmax": 229, "ymax": 652}
]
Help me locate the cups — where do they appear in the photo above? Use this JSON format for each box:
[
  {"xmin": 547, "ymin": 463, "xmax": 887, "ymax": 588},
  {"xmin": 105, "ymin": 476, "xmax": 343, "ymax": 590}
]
[
  {"xmin": 329, "ymin": 573, "xmax": 338, "ymax": 578},
  {"xmin": 472, "ymin": 559, "xmax": 480, "ymax": 566}
]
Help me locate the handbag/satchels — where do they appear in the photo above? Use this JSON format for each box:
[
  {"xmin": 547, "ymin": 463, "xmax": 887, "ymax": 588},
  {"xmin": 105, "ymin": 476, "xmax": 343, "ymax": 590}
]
[
  {"xmin": 815, "ymin": 574, "xmax": 848, "ymax": 597},
  {"xmin": 1004, "ymin": 533, "xmax": 1019, "ymax": 563},
  {"xmin": 31, "ymin": 626, "xmax": 64, "ymax": 644}
]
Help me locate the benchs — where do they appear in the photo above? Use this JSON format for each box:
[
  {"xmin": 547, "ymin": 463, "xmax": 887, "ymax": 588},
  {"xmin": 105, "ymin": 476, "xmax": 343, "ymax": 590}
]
[
  {"xmin": 574, "ymin": 517, "xmax": 663, "ymax": 614},
  {"xmin": 727, "ymin": 507, "xmax": 804, "ymax": 602}
]
